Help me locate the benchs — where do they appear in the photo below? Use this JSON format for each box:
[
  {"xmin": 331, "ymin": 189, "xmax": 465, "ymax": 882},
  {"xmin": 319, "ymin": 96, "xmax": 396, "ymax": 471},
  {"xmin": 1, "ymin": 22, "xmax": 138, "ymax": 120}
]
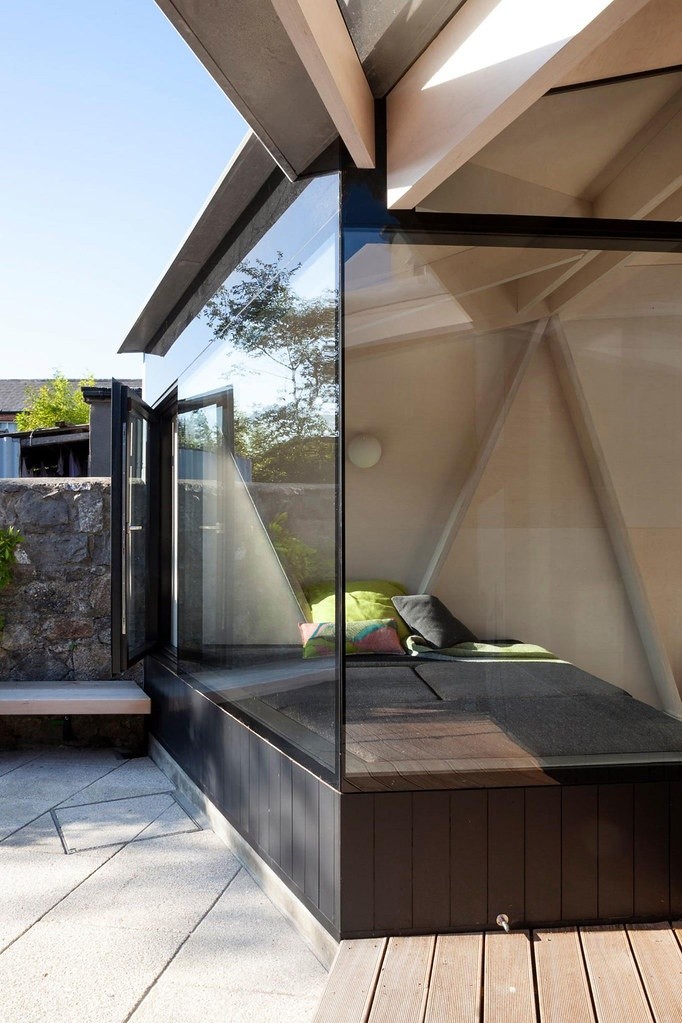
[{"xmin": 0, "ymin": 681, "xmax": 151, "ymax": 759}]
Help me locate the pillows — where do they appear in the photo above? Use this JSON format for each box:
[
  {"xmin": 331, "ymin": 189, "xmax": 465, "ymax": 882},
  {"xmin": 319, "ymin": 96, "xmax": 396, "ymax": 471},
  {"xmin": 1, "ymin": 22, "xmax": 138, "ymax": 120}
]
[
  {"xmin": 392, "ymin": 594, "xmax": 477, "ymax": 648},
  {"xmin": 304, "ymin": 580, "xmax": 410, "ymax": 641},
  {"xmin": 298, "ymin": 617, "xmax": 406, "ymax": 659}
]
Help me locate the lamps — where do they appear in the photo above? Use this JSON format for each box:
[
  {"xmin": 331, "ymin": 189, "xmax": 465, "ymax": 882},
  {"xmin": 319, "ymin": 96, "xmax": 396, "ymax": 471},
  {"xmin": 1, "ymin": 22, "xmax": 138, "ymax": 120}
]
[{"xmin": 348, "ymin": 434, "xmax": 383, "ymax": 469}]
